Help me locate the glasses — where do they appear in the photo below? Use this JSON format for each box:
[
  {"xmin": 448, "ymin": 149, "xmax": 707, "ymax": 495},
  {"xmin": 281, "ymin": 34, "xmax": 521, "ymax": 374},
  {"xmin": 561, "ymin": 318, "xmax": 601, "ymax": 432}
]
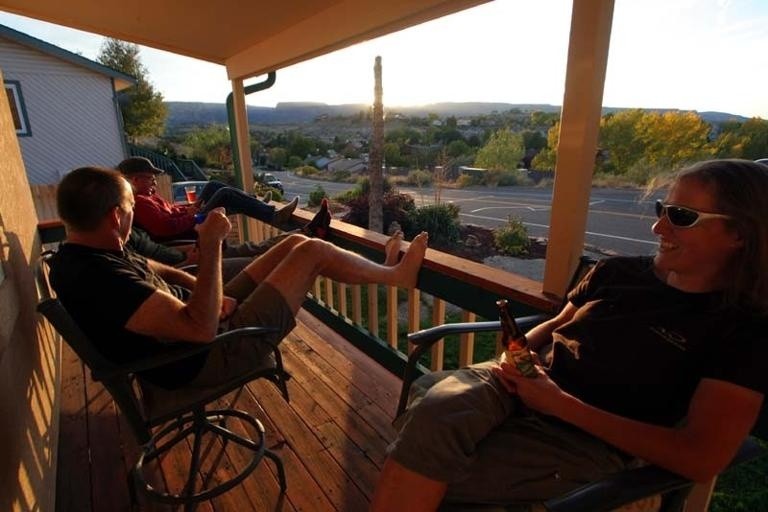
[{"xmin": 655, "ymin": 199, "xmax": 731, "ymax": 229}]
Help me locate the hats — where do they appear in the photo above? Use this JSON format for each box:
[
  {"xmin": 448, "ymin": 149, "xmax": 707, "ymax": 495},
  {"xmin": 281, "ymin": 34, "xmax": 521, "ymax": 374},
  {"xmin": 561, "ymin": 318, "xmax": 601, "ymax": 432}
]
[{"xmin": 118, "ymin": 158, "xmax": 165, "ymax": 174}]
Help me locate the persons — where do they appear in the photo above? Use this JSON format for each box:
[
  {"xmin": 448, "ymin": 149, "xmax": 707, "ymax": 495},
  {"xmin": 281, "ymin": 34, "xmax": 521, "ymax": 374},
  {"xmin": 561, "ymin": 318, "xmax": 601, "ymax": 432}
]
[
  {"xmin": 371, "ymin": 156, "xmax": 766, "ymax": 511},
  {"xmin": 112, "ymin": 155, "xmax": 301, "ymax": 239},
  {"xmin": 46, "ymin": 164, "xmax": 429, "ymax": 391},
  {"xmin": 122, "ymin": 197, "xmax": 333, "ymax": 287}
]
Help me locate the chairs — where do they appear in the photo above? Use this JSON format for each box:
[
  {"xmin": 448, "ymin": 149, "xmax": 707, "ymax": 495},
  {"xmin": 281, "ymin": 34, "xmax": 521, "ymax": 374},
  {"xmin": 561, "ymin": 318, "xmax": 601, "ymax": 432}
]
[
  {"xmin": 392, "ymin": 256, "xmax": 695, "ymax": 512},
  {"xmin": 156, "ymin": 238, "xmax": 228, "ymax": 246},
  {"xmin": 36, "ymin": 249, "xmax": 292, "ymax": 512}
]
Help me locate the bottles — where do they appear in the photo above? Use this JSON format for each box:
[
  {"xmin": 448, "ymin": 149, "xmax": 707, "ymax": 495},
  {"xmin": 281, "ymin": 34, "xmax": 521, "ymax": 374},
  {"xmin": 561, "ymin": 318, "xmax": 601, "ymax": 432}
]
[{"xmin": 495, "ymin": 298, "xmax": 536, "ymax": 381}]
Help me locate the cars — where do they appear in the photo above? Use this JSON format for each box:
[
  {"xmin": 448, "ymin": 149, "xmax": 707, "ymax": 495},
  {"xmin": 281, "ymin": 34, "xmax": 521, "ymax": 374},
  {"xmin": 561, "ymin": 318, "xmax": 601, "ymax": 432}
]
[{"xmin": 258, "ymin": 173, "xmax": 283, "ymax": 196}]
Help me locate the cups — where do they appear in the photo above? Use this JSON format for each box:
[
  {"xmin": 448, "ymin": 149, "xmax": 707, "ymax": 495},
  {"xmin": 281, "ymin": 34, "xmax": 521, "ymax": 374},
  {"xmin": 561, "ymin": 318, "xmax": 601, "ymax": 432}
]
[
  {"xmin": 184, "ymin": 186, "xmax": 198, "ymax": 204},
  {"xmin": 192, "ymin": 211, "xmax": 211, "ymax": 248}
]
